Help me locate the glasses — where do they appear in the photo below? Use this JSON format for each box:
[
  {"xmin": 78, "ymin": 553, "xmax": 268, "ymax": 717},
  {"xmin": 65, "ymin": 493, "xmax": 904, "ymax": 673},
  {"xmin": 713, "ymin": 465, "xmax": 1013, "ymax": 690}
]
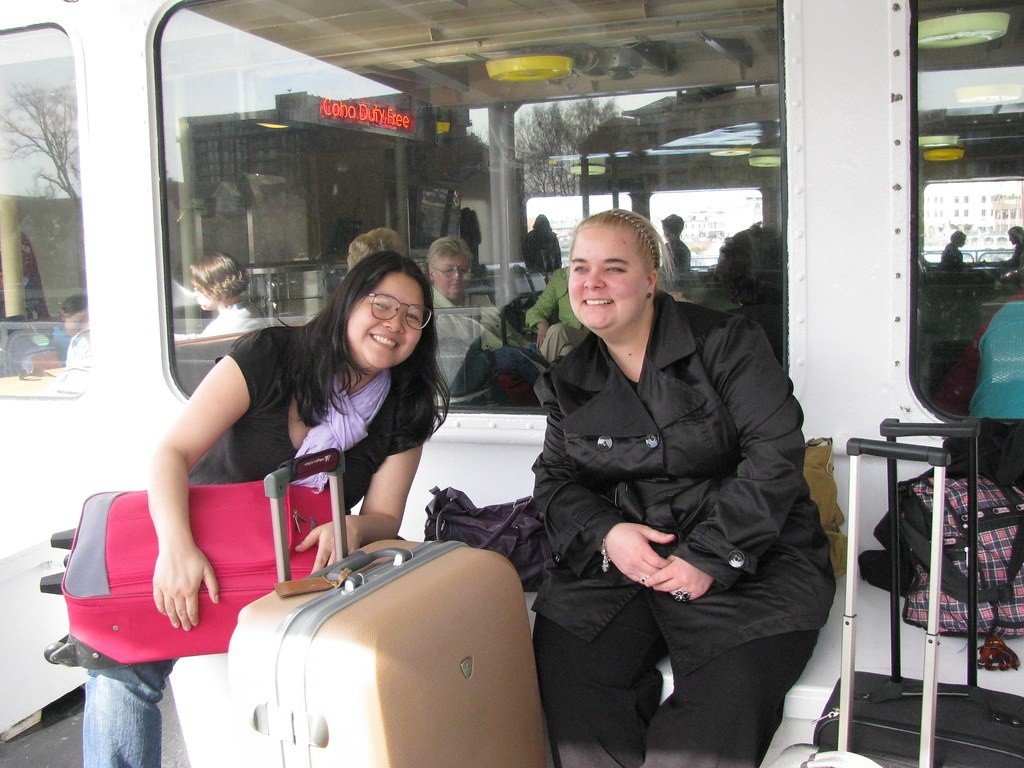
[
  {"xmin": 433, "ymin": 267, "xmax": 471, "ymax": 277},
  {"xmin": 365, "ymin": 293, "xmax": 433, "ymax": 331}
]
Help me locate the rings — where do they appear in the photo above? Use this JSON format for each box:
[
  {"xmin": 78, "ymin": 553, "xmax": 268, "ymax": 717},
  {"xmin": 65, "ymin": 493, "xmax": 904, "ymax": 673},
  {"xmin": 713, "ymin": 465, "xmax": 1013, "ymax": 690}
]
[
  {"xmin": 673, "ymin": 590, "xmax": 690, "ymax": 602},
  {"xmin": 640, "ymin": 575, "xmax": 650, "ymax": 583}
]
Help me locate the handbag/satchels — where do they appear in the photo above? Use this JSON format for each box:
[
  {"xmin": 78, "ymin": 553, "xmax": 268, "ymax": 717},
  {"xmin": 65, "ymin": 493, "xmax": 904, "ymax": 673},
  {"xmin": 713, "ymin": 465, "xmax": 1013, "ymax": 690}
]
[
  {"xmin": 424, "ymin": 485, "xmax": 552, "ymax": 592},
  {"xmin": 804, "ymin": 436, "xmax": 850, "ymax": 578},
  {"xmin": 858, "ymin": 415, "xmax": 1024, "ymax": 670}
]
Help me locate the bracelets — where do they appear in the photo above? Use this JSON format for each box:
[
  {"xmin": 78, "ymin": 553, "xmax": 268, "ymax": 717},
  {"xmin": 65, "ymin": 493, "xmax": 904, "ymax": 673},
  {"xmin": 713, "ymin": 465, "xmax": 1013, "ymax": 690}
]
[{"xmin": 601, "ymin": 537, "xmax": 610, "ymax": 572}]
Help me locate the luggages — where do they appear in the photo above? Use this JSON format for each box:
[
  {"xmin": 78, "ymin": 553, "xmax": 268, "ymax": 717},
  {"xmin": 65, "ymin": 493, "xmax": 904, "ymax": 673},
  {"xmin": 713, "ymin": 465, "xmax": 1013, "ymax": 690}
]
[
  {"xmin": 224, "ymin": 447, "xmax": 545, "ymax": 768},
  {"xmin": 812, "ymin": 418, "xmax": 1024, "ymax": 768},
  {"xmin": 766, "ymin": 437, "xmax": 957, "ymax": 768},
  {"xmin": 40, "ymin": 480, "xmax": 347, "ymax": 669}
]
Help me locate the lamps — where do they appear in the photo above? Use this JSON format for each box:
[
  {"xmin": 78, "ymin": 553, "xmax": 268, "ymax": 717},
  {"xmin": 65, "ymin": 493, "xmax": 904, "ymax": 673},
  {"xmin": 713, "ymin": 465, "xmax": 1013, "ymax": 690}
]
[
  {"xmin": 711, "ymin": 147, "xmax": 752, "ymax": 157},
  {"xmin": 918, "ymin": 133, "xmax": 956, "ymax": 146},
  {"xmin": 569, "ymin": 156, "xmax": 607, "ymax": 175},
  {"xmin": 748, "ymin": 154, "xmax": 782, "ymax": 166},
  {"xmin": 957, "ymin": 79, "xmax": 1021, "ymax": 109},
  {"xmin": 917, "ymin": 11, "xmax": 1011, "ymax": 53},
  {"xmin": 486, "ymin": 56, "xmax": 576, "ymax": 82},
  {"xmin": 436, "ymin": 111, "xmax": 453, "ymax": 132},
  {"xmin": 924, "ymin": 148, "xmax": 964, "ymax": 163}
]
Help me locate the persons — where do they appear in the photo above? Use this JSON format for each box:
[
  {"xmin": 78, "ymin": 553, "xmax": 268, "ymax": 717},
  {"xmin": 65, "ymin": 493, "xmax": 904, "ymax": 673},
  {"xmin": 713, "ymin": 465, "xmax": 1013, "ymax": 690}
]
[
  {"xmin": 529, "ymin": 209, "xmax": 837, "ymax": 768},
  {"xmin": 941, "ymin": 230, "xmax": 967, "ymax": 263},
  {"xmin": 427, "ymin": 235, "xmax": 531, "ymax": 351},
  {"xmin": 998, "ymin": 226, "xmax": 1024, "ymax": 269},
  {"xmin": 526, "ymin": 266, "xmax": 591, "ymax": 363},
  {"xmin": 347, "ymin": 228, "xmax": 408, "ymax": 272},
  {"xmin": 523, "ymin": 214, "xmax": 562, "ymax": 272},
  {"xmin": 174, "ymin": 252, "xmax": 271, "ymax": 341},
  {"xmin": 937, "ymin": 287, "xmax": 1024, "ymax": 461},
  {"xmin": 83, "ymin": 251, "xmax": 450, "ymax": 768},
  {"xmin": 52, "ymin": 294, "xmax": 87, "ymax": 368},
  {"xmin": 661, "ymin": 214, "xmax": 691, "ymax": 271}
]
